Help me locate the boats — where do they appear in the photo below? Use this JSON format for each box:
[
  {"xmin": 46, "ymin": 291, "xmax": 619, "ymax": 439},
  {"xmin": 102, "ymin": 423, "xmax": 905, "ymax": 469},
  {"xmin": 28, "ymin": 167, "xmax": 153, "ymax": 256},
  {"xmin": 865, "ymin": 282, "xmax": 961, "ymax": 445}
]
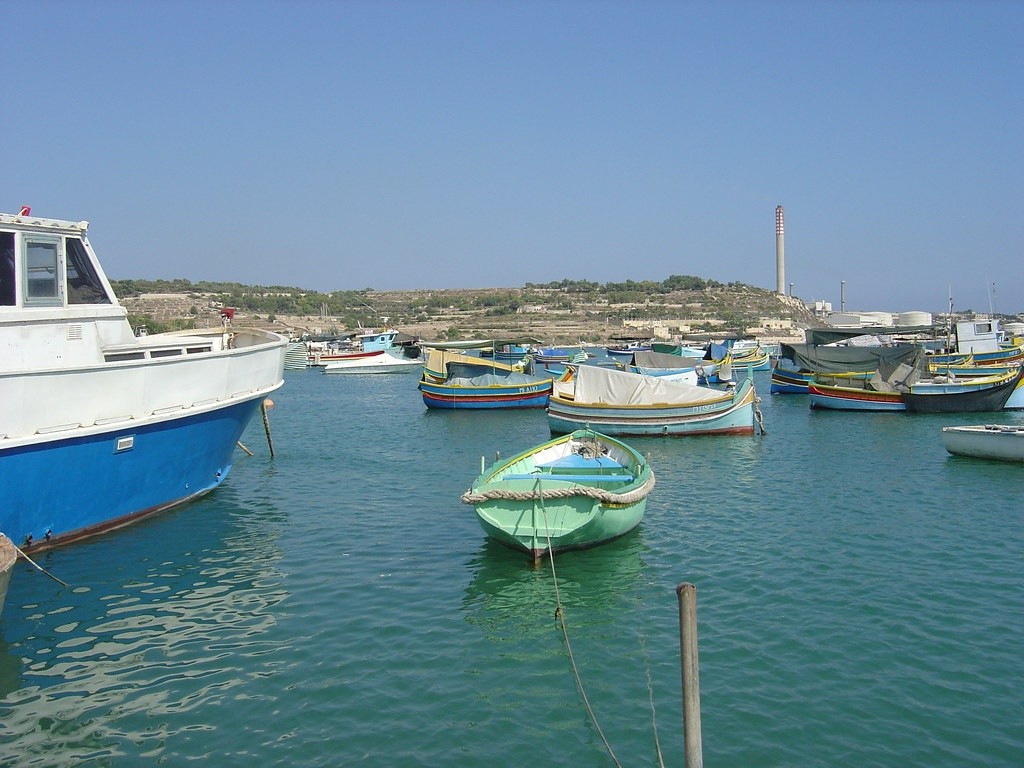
[
  {"xmin": 462, "ymin": 423, "xmax": 656, "ymax": 565},
  {"xmin": 544, "ymin": 364, "xmax": 767, "ymax": 439},
  {"xmin": 0, "ymin": 206, "xmax": 288, "ymax": 544},
  {"xmin": 306, "ymin": 296, "xmax": 1024, "ymax": 413},
  {"xmin": 940, "ymin": 423, "xmax": 1023, "ymax": 464}
]
